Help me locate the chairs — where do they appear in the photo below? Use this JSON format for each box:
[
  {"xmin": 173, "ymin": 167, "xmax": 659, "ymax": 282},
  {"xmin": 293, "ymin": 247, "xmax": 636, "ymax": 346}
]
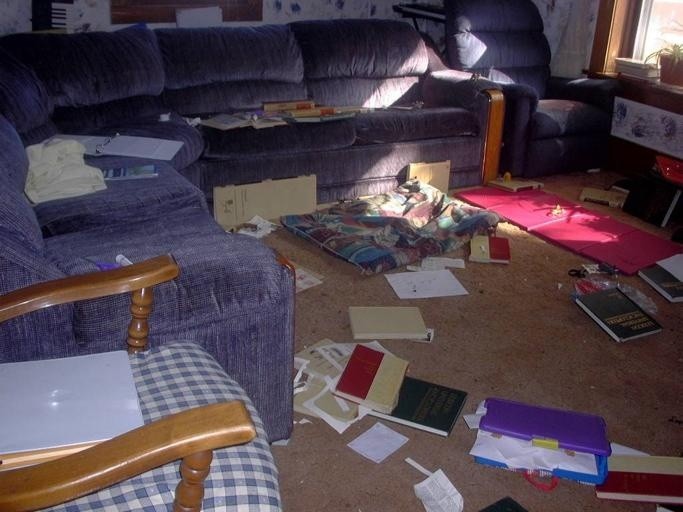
[
  {"xmin": 0, "ymin": 251, "xmax": 282, "ymax": 512},
  {"xmin": 440, "ymin": 0, "xmax": 617, "ymax": 180}
]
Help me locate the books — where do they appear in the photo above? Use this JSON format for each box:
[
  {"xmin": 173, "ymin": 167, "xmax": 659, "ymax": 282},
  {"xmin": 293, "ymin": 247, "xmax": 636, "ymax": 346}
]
[
  {"xmin": 596, "ymin": 456, "xmax": 683, "ymax": 505},
  {"xmin": 638, "ymin": 267, "xmax": 683, "ymax": 303},
  {"xmin": 102, "ymin": 165, "xmax": 158, "ymax": 180},
  {"xmin": 0, "ymin": 350, "xmax": 145, "ymax": 475},
  {"xmin": 576, "ymin": 287, "xmax": 663, "ymax": 344},
  {"xmin": 335, "ymin": 343, "xmax": 410, "ymax": 415},
  {"xmin": 367, "ymin": 375, "xmax": 468, "ymax": 437},
  {"xmin": 580, "ymin": 187, "xmax": 627, "ymax": 208},
  {"xmin": 349, "ymin": 306, "xmax": 427, "ymax": 342},
  {"xmin": 204, "ymin": 98, "xmax": 356, "ymax": 130},
  {"xmin": 615, "ymin": 56, "xmax": 660, "ymax": 85},
  {"xmin": 487, "ymin": 179, "xmax": 545, "ymax": 192},
  {"xmin": 469, "ymin": 234, "xmax": 510, "ymax": 266},
  {"xmin": 407, "ymin": 160, "xmax": 450, "ymax": 196}
]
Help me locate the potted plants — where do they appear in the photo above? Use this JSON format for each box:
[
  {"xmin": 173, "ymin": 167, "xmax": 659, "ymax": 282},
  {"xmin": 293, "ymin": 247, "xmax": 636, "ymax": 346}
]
[{"xmin": 643, "ymin": 39, "xmax": 683, "ymax": 90}]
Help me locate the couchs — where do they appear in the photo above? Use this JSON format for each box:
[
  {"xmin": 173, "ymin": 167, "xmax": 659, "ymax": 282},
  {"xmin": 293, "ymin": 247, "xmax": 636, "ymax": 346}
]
[{"xmin": 0, "ymin": 18, "xmax": 506, "ymax": 445}]
